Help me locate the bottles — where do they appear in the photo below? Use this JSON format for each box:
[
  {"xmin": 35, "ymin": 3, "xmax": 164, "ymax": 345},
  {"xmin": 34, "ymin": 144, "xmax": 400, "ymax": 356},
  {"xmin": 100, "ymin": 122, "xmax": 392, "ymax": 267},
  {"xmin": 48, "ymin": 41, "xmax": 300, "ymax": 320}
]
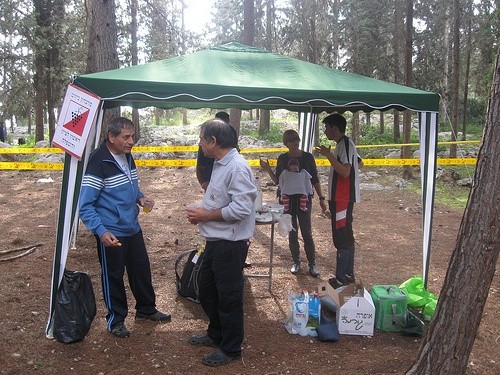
[{"xmin": 299, "ymin": 290, "xmax": 320, "ymax": 316}]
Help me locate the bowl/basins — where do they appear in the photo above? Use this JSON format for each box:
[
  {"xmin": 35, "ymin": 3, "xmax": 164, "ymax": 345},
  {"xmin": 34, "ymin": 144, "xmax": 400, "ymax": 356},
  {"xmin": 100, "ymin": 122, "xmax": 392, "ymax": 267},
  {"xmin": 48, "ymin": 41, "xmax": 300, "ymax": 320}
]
[{"xmin": 255, "ymin": 201, "xmax": 284, "ymax": 223}]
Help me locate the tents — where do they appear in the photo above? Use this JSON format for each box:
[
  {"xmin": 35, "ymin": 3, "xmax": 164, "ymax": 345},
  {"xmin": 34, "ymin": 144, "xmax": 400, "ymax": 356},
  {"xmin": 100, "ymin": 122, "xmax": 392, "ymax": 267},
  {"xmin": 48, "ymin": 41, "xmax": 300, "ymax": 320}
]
[{"xmin": 45, "ymin": 42, "xmax": 440, "ymax": 340}]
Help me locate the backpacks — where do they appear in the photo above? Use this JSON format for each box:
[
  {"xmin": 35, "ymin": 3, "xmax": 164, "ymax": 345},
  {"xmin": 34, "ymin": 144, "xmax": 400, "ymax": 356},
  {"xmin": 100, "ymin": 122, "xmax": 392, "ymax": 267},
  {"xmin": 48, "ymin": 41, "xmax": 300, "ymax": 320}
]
[{"xmin": 175, "ymin": 249, "xmax": 204, "ymax": 304}]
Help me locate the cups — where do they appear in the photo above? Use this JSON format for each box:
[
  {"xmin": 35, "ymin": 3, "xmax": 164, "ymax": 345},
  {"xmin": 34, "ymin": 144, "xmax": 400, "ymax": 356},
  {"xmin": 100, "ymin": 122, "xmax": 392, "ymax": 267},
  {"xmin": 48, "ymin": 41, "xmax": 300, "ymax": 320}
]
[
  {"xmin": 143, "ymin": 200, "xmax": 152, "ymax": 213},
  {"xmin": 187, "ymin": 203, "xmax": 198, "ymax": 208}
]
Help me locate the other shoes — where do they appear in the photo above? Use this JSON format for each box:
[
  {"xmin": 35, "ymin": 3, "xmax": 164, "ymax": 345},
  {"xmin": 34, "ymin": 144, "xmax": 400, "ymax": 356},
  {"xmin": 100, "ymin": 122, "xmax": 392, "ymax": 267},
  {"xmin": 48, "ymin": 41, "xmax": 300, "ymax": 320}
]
[
  {"xmin": 190, "ymin": 333, "xmax": 219, "ymax": 348},
  {"xmin": 309, "ymin": 266, "xmax": 320, "ymax": 276},
  {"xmin": 329, "ymin": 278, "xmax": 345, "ymax": 289},
  {"xmin": 202, "ymin": 349, "xmax": 241, "ymax": 366},
  {"xmin": 290, "ymin": 263, "xmax": 299, "ymax": 273}
]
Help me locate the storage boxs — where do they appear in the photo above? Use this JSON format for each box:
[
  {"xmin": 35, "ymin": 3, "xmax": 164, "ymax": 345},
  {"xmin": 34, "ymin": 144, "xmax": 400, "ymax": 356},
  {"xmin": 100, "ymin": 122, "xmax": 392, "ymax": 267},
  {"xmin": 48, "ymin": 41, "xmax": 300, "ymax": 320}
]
[{"xmin": 318, "ymin": 278, "xmax": 407, "ymax": 336}]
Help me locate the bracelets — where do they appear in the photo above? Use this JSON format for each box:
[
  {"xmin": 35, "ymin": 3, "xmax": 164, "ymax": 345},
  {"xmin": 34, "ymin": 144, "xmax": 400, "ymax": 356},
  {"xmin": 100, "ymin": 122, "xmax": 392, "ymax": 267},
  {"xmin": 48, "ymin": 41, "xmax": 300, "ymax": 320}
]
[{"xmin": 319, "ymin": 196, "xmax": 325, "ymax": 200}]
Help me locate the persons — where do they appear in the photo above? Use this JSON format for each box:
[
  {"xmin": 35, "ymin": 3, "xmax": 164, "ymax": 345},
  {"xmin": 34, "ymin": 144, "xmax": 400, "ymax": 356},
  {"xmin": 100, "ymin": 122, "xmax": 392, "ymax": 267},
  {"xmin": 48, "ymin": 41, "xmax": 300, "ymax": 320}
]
[
  {"xmin": 186, "ymin": 118, "xmax": 258, "ymax": 366},
  {"xmin": 196, "ymin": 112, "xmax": 239, "ymax": 192},
  {"xmin": 315, "ymin": 115, "xmax": 364, "ymax": 289},
  {"xmin": 281, "ymin": 158, "xmax": 308, "ymax": 214},
  {"xmin": 79, "ymin": 117, "xmax": 171, "ymax": 336},
  {"xmin": 260, "ymin": 129, "xmax": 327, "ymax": 276}
]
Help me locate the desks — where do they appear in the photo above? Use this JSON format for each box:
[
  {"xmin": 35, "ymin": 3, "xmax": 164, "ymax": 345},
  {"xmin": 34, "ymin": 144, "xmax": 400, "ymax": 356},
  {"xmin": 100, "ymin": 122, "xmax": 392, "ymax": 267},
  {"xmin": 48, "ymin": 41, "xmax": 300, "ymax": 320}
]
[{"xmin": 243, "ymin": 211, "xmax": 277, "ymax": 292}]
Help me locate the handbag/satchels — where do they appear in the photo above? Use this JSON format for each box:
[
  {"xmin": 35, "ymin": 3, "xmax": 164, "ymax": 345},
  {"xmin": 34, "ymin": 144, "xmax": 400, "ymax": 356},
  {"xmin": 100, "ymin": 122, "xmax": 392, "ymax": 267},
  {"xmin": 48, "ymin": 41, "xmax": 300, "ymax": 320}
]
[{"xmin": 398, "ymin": 276, "xmax": 436, "ymax": 308}]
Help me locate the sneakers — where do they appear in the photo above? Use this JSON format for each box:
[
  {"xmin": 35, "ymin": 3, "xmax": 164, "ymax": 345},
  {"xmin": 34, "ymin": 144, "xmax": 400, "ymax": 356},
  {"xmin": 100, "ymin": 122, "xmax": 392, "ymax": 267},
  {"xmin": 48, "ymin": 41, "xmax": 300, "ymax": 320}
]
[
  {"xmin": 135, "ymin": 311, "xmax": 171, "ymax": 322},
  {"xmin": 112, "ymin": 324, "xmax": 130, "ymax": 338}
]
